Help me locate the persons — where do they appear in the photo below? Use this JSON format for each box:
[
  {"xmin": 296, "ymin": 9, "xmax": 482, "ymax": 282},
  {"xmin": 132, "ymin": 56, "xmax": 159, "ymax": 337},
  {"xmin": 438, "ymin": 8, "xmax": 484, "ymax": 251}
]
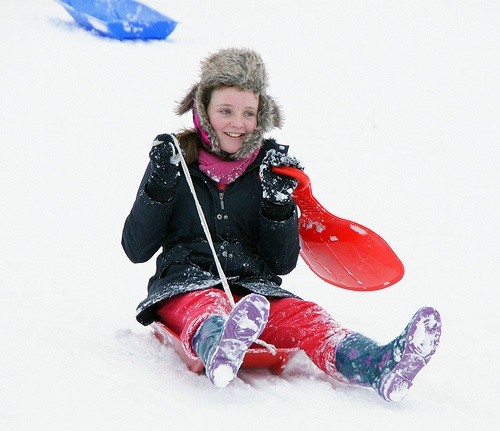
[{"xmin": 120, "ymin": 45, "xmax": 441, "ymax": 403}]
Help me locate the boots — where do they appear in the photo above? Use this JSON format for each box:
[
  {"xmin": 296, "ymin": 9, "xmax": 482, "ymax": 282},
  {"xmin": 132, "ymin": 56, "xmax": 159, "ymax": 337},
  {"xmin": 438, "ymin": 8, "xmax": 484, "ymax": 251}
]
[
  {"xmin": 336, "ymin": 307, "xmax": 441, "ymax": 403},
  {"xmin": 192, "ymin": 293, "xmax": 270, "ymax": 387}
]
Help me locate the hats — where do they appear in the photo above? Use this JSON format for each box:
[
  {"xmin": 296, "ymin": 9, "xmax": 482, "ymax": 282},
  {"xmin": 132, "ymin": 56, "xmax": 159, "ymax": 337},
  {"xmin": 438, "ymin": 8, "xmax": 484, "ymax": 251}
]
[{"xmin": 177, "ymin": 50, "xmax": 280, "ymax": 162}]
